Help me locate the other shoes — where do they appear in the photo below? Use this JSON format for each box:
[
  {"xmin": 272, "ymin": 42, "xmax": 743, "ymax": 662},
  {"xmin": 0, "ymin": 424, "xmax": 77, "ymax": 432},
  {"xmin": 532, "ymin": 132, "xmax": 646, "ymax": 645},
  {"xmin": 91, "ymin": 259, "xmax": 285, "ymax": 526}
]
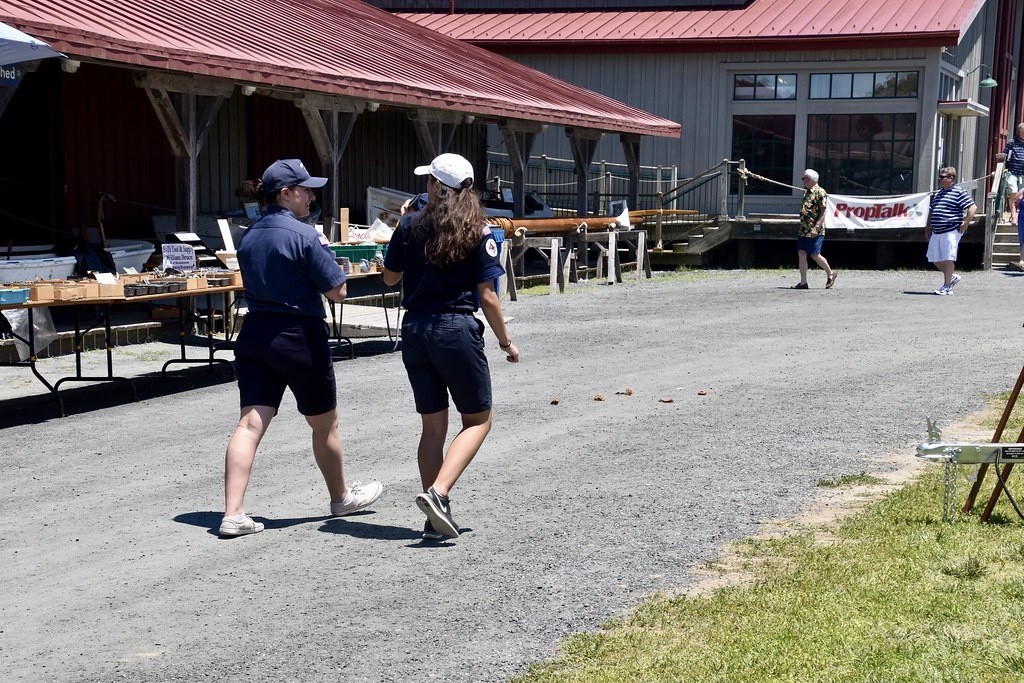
[
  {"xmin": 825, "ymin": 272, "xmax": 837, "ymax": 289},
  {"xmin": 1009, "ymin": 261, "xmax": 1024, "ymax": 271},
  {"xmin": 789, "ymin": 283, "xmax": 808, "ymax": 288}
]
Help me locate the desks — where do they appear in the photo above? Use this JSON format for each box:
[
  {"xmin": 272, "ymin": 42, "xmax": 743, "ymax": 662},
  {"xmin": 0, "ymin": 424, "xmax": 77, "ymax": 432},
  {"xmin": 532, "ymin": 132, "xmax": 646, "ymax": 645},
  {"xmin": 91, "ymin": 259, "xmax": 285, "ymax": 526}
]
[
  {"xmin": 328, "ymin": 264, "xmax": 404, "ymax": 357},
  {"xmin": 0, "ymin": 274, "xmax": 243, "ymax": 418}
]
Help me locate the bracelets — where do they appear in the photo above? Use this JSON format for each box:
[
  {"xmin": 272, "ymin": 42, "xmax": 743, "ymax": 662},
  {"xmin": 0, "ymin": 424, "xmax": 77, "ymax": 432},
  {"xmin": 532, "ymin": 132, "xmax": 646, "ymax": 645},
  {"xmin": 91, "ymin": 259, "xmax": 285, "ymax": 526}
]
[
  {"xmin": 499, "ymin": 339, "xmax": 512, "ymax": 348},
  {"xmin": 815, "ymin": 224, "xmax": 820, "ymax": 229}
]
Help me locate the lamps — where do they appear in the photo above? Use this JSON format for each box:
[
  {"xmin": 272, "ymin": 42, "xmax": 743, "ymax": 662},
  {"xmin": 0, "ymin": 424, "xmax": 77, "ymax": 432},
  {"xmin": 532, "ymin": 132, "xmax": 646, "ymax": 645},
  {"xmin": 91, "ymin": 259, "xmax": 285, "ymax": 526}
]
[
  {"xmin": 240, "ymin": 85, "xmax": 256, "ymax": 96},
  {"xmin": 369, "ymin": 103, "xmax": 379, "ymax": 112},
  {"xmin": 967, "ymin": 64, "xmax": 998, "ymax": 88},
  {"xmin": 61, "ymin": 60, "xmax": 81, "ymax": 73},
  {"xmin": 465, "ymin": 115, "xmax": 475, "ymax": 124}
]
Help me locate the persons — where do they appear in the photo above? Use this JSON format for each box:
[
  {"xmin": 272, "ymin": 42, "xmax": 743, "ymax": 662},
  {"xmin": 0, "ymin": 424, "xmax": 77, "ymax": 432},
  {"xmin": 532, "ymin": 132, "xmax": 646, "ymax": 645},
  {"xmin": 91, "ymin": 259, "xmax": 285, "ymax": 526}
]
[
  {"xmin": 220, "ymin": 159, "xmax": 382, "ymax": 534},
  {"xmin": 925, "ymin": 167, "xmax": 977, "ymax": 295},
  {"xmin": 1015, "ymin": 187, "xmax": 1024, "ymax": 271},
  {"xmin": 791, "ymin": 169, "xmax": 837, "ymax": 289},
  {"xmin": 384, "ymin": 153, "xmax": 519, "ymax": 538},
  {"xmin": 1003, "ymin": 123, "xmax": 1024, "ymax": 224}
]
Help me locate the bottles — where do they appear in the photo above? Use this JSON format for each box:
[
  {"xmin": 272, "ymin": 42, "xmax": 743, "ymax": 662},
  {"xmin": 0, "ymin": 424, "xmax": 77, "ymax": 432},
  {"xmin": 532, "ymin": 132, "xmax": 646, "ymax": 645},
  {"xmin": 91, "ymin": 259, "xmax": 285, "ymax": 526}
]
[
  {"xmin": 415, "ymin": 190, "xmax": 429, "ymax": 212},
  {"xmin": 360, "ymin": 258, "xmax": 372, "ymax": 273}
]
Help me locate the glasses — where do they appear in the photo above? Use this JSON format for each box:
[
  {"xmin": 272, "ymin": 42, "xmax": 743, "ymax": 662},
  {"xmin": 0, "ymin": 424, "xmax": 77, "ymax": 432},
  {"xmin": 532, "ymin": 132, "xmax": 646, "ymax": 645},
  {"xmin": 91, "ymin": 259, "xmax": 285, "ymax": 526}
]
[
  {"xmin": 802, "ymin": 178, "xmax": 807, "ymax": 180},
  {"xmin": 938, "ymin": 175, "xmax": 951, "ymax": 179}
]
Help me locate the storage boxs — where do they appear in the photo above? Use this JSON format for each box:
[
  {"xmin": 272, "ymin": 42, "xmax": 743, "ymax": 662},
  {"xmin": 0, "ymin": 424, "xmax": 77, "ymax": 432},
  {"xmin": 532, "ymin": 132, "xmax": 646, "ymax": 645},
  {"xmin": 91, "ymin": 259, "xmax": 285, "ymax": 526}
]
[
  {"xmin": 0, "ymin": 270, "xmax": 242, "ymax": 304},
  {"xmin": 329, "ymin": 243, "xmax": 386, "ymax": 262}
]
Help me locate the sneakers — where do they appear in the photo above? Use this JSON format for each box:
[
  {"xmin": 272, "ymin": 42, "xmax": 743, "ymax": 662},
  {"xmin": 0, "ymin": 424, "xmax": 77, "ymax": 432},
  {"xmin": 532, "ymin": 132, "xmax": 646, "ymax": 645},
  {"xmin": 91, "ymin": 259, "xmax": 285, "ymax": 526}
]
[
  {"xmin": 949, "ymin": 274, "xmax": 961, "ymax": 286},
  {"xmin": 422, "ymin": 519, "xmax": 444, "ymax": 539},
  {"xmin": 219, "ymin": 512, "xmax": 264, "ymax": 536},
  {"xmin": 415, "ymin": 486, "xmax": 460, "ymax": 538},
  {"xmin": 933, "ymin": 286, "xmax": 953, "ymax": 295},
  {"xmin": 330, "ymin": 480, "xmax": 383, "ymax": 516}
]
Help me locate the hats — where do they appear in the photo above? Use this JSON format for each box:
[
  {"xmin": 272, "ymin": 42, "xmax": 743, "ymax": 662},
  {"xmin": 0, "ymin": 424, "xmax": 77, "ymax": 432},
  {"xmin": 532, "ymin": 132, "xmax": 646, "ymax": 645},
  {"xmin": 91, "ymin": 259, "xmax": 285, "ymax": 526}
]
[
  {"xmin": 262, "ymin": 158, "xmax": 328, "ymax": 194},
  {"xmin": 414, "ymin": 152, "xmax": 474, "ymax": 189}
]
